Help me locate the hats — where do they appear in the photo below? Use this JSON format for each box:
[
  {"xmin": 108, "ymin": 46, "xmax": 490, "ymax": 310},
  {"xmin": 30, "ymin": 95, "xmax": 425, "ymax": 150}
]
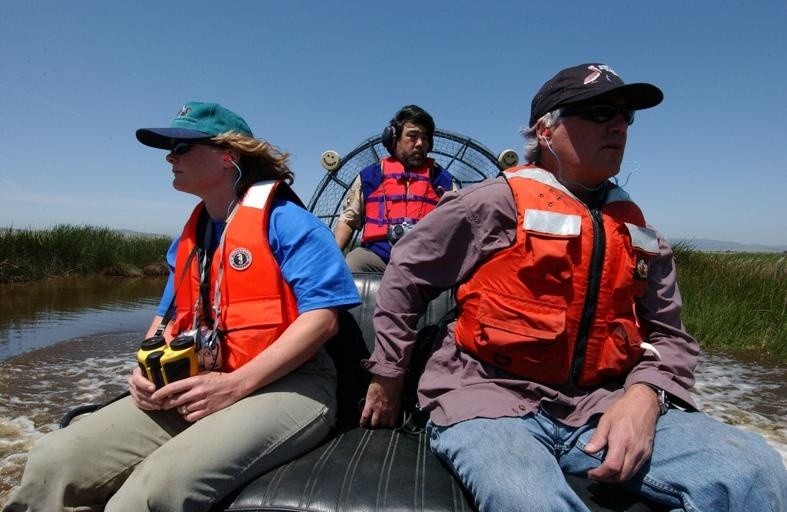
[
  {"xmin": 529, "ymin": 63, "xmax": 664, "ymax": 127},
  {"xmin": 136, "ymin": 101, "xmax": 253, "ymax": 150}
]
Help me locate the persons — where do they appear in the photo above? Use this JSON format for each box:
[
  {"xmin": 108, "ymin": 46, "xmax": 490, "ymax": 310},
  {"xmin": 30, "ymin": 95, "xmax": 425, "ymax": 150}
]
[
  {"xmin": 358, "ymin": 62, "xmax": 787, "ymax": 512},
  {"xmin": 0, "ymin": 100, "xmax": 363, "ymax": 512},
  {"xmin": 333, "ymin": 104, "xmax": 460, "ymax": 273}
]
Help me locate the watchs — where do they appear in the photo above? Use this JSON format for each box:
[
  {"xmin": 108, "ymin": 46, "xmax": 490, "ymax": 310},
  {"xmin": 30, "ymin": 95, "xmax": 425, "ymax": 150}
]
[{"xmin": 649, "ymin": 384, "xmax": 672, "ymax": 417}]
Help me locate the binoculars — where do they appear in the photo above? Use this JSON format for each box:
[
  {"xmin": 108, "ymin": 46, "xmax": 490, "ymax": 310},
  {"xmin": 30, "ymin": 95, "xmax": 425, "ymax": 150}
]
[{"xmin": 133, "ymin": 333, "xmax": 200, "ymax": 400}]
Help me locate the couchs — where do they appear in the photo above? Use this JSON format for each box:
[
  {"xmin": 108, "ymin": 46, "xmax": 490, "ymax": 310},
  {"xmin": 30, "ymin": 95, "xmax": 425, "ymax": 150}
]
[{"xmin": 224, "ymin": 268, "xmax": 673, "ymax": 512}]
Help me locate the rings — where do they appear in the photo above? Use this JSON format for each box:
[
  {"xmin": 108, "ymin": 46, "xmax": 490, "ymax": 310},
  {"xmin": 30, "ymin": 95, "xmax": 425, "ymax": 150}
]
[{"xmin": 183, "ymin": 404, "xmax": 190, "ymax": 416}]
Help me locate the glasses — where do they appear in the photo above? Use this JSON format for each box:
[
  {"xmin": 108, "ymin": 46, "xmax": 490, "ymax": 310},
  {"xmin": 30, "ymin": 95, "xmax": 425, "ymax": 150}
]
[
  {"xmin": 173, "ymin": 142, "xmax": 191, "ymax": 155},
  {"xmin": 560, "ymin": 106, "xmax": 634, "ymax": 126}
]
